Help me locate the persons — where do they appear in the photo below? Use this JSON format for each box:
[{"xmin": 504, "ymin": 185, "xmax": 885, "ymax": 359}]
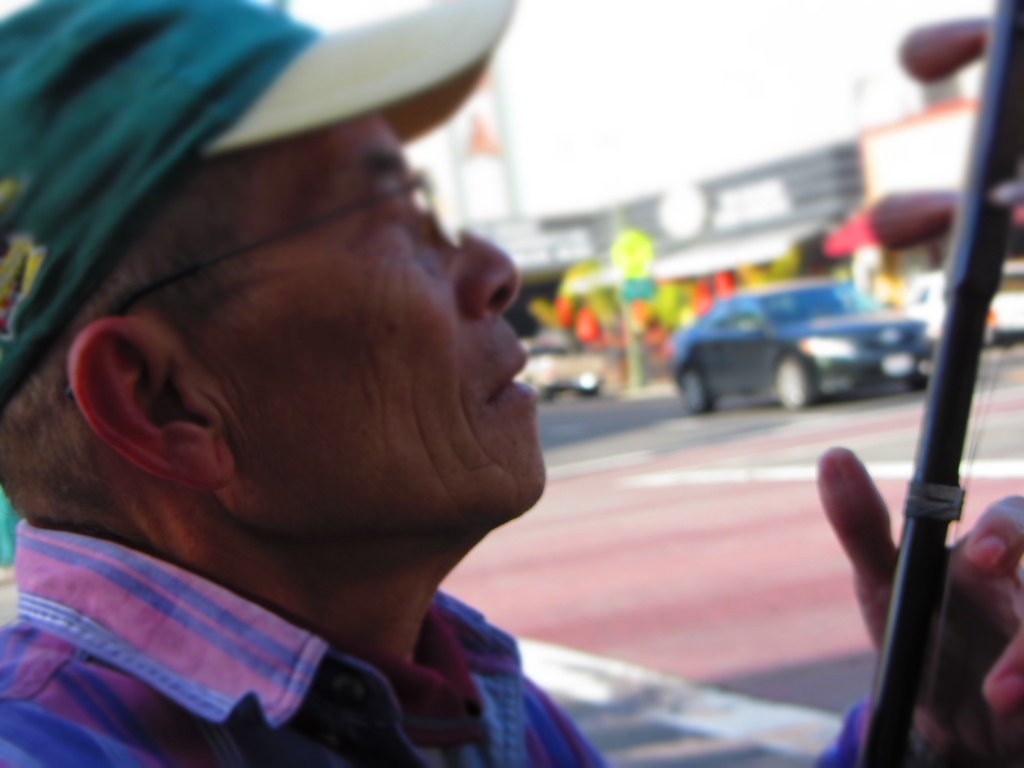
[{"xmin": 0, "ymin": 0, "xmax": 1024, "ymax": 768}]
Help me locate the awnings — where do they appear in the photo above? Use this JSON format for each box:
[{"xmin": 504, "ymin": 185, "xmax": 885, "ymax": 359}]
[{"xmin": 649, "ymin": 217, "xmax": 819, "ymax": 277}]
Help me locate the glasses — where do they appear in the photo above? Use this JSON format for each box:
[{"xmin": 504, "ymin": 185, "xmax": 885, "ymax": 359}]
[{"xmin": 60, "ymin": 170, "xmax": 444, "ymax": 399}]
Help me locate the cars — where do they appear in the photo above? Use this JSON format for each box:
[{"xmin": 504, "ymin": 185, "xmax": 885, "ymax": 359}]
[
  {"xmin": 668, "ymin": 282, "xmax": 935, "ymax": 417},
  {"xmin": 515, "ymin": 333, "xmax": 610, "ymax": 401},
  {"xmin": 901, "ymin": 256, "xmax": 1024, "ymax": 352}
]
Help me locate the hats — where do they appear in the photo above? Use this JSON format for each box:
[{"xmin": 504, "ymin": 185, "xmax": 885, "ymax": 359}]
[{"xmin": 0, "ymin": 0, "xmax": 513, "ymax": 397}]
